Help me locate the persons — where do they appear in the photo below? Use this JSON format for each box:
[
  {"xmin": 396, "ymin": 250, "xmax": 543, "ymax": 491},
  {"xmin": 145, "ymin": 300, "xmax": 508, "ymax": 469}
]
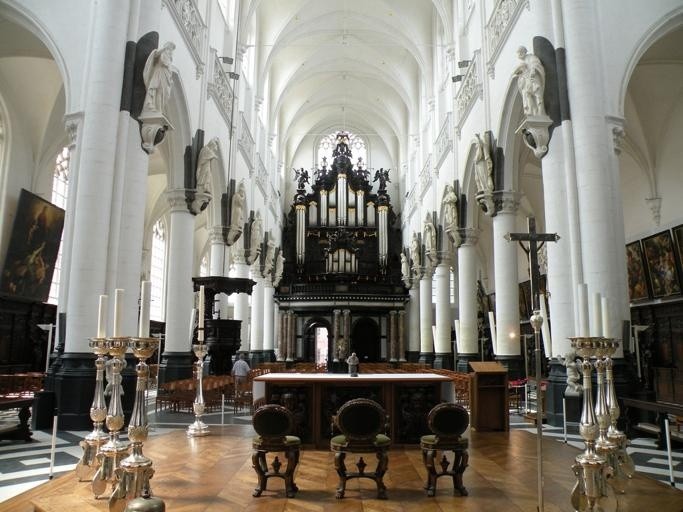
[
  {"xmin": 510, "ymin": 44, "xmax": 547, "ymax": 118},
  {"xmin": 194, "ymin": 138, "xmax": 218, "ymax": 196},
  {"xmin": 231, "ymin": 353, "xmax": 252, "ymax": 394},
  {"xmin": 292, "ymin": 166, "xmax": 391, "ymax": 191},
  {"xmin": 399, "ymin": 185, "xmax": 457, "ymax": 276},
  {"xmin": 141, "ymin": 40, "xmax": 177, "ymax": 116},
  {"xmin": 472, "ymin": 132, "xmax": 489, "ymax": 192},
  {"xmin": 557, "ymin": 351, "xmax": 581, "ymax": 392},
  {"xmin": 6, "ymin": 205, "xmax": 49, "ymax": 293},
  {"xmin": 346, "ymin": 352, "xmax": 359, "ymax": 374},
  {"xmin": 231, "ymin": 186, "xmax": 286, "ymax": 278}
]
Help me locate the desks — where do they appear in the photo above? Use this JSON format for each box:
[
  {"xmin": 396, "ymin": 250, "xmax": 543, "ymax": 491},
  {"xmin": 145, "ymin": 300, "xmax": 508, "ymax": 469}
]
[
  {"xmin": 0, "ymin": 395, "xmax": 38, "ymax": 443},
  {"xmin": 252, "ymin": 371, "xmax": 456, "ymax": 451}
]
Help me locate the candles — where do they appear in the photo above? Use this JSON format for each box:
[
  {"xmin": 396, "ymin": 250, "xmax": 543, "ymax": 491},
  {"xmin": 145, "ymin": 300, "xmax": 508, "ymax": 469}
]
[
  {"xmin": 432, "ymin": 325, "xmax": 436, "ymax": 343},
  {"xmin": 454, "ymin": 319, "xmax": 460, "ymax": 347},
  {"xmin": 114, "ymin": 288, "xmax": 124, "ymax": 337},
  {"xmin": 138, "ymin": 281, "xmax": 152, "ymax": 338},
  {"xmin": 488, "ymin": 311, "xmax": 496, "ymax": 354},
  {"xmin": 197, "ymin": 285, "xmax": 205, "ymax": 341},
  {"xmin": 577, "ymin": 282, "xmax": 611, "ymax": 340},
  {"xmin": 537, "ymin": 293, "xmax": 552, "ymax": 358},
  {"xmin": 97, "ymin": 295, "xmax": 108, "ymax": 338}
]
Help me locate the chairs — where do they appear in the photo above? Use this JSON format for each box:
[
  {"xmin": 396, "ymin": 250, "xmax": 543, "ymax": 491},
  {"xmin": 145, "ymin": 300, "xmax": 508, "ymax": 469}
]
[
  {"xmin": 329, "ymin": 398, "xmax": 395, "ymax": 500},
  {"xmin": 250, "ymin": 404, "xmax": 302, "ymax": 499},
  {"xmin": 419, "ymin": 404, "xmax": 472, "ymax": 497}
]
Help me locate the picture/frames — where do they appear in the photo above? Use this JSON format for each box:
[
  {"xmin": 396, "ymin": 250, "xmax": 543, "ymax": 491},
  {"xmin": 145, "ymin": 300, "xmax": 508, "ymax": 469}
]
[
  {"xmin": 641, "ymin": 230, "xmax": 682, "ymax": 299},
  {"xmin": 0, "ymin": 187, "xmax": 66, "ymax": 303},
  {"xmin": 626, "ymin": 240, "xmax": 650, "ymax": 302},
  {"xmin": 482, "ymin": 274, "xmax": 548, "ymax": 341},
  {"xmin": 671, "ymin": 224, "xmax": 683, "ymax": 271}
]
